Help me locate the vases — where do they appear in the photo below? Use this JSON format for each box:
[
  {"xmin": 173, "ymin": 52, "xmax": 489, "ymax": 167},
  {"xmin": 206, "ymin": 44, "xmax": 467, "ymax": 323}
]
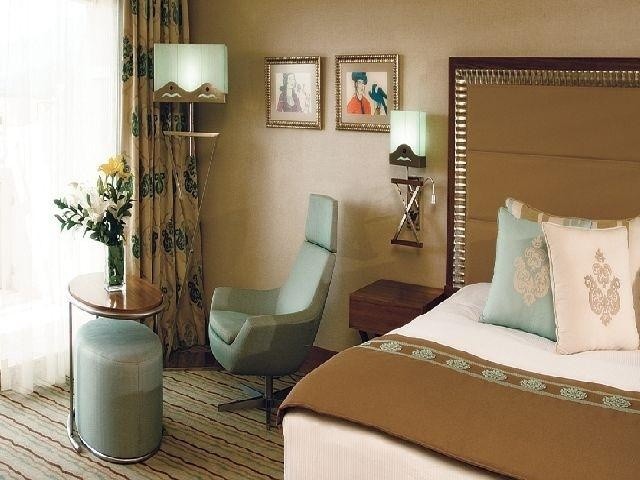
[{"xmin": 104, "ymin": 240, "xmax": 127, "ymax": 293}]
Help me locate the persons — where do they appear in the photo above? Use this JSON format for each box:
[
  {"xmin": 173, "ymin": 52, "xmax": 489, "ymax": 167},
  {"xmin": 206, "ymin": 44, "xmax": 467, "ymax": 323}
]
[
  {"xmin": 277, "ymin": 72, "xmax": 303, "ymax": 112},
  {"xmin": 347, "ymin": 71, "xmax": 371, "ymax": 115}
]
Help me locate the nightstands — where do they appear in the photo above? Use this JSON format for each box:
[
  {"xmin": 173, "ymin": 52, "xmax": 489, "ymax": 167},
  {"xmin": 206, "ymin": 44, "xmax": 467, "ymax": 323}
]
[{"xmin": 345, "ymin": 277, "xmax": 446, "ymax": 345}]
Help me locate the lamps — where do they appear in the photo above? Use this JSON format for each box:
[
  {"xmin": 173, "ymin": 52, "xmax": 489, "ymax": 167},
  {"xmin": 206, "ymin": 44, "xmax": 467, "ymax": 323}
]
[
  {"xmin": 144, "ymin": 40, "xmax": 230, "ymax": 372},
  {"xmin": 387, "ymin": 108, "xmax": 430, "ymax": 231}
]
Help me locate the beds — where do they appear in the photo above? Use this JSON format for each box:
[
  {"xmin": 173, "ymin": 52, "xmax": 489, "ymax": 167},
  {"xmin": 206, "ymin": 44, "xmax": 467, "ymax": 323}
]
[{"xmin": 268, "ymin": 53, "xmax": 640, "ymax": 480}]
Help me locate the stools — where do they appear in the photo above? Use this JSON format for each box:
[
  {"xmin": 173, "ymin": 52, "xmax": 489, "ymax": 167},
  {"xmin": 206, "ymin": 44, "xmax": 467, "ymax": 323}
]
[{"xmin": 71, "ymin": 316, "xmax": 167, "ymax": 464}]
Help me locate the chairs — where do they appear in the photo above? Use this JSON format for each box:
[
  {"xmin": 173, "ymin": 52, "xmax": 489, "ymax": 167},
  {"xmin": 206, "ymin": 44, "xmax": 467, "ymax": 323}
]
[{"xmin": 204, "ymin": 189, "xmax": 343, "ymax": 435}]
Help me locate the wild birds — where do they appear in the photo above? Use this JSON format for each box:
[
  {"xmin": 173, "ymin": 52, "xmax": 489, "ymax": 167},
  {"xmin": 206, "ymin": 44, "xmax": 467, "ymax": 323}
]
[{"xmin": 367, "ymin": 83, "xmax": 387, "ymax": 114}]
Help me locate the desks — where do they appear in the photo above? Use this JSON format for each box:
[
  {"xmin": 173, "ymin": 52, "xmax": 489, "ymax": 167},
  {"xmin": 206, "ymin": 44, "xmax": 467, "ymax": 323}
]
[{"xmin": 63, "ymin": 270, "xmax": 171, "ymax": 459}]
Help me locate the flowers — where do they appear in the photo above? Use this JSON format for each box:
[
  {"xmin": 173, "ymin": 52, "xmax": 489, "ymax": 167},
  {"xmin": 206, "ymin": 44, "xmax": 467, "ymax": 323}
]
[{"xmin": 50, "ymin": 153, "xmax": 138, "ymax": 286}]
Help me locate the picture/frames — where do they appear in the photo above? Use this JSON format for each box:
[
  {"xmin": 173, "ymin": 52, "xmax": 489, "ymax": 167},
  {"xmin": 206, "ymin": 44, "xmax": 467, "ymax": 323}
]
[
  {"xmin": 332, "ymin": 53, "xmax": 402, "ymax": 135},
  {"xmin": 263, "ymin": 54, "xmax": 325, "ymax": 131}
]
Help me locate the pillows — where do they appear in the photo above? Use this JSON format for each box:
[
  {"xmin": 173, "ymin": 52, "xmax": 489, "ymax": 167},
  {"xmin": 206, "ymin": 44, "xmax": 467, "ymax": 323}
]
[
  {"xmin": 536, "ymin": 217, "xmax": 640, "ymax": 357},
  {"xmin": 504, "ymin": 196, "xmax": 639, "ymax": 352},
  {"xmin": 476, "ymin": 205, "xmax": 556, "ymax": 345}
]
